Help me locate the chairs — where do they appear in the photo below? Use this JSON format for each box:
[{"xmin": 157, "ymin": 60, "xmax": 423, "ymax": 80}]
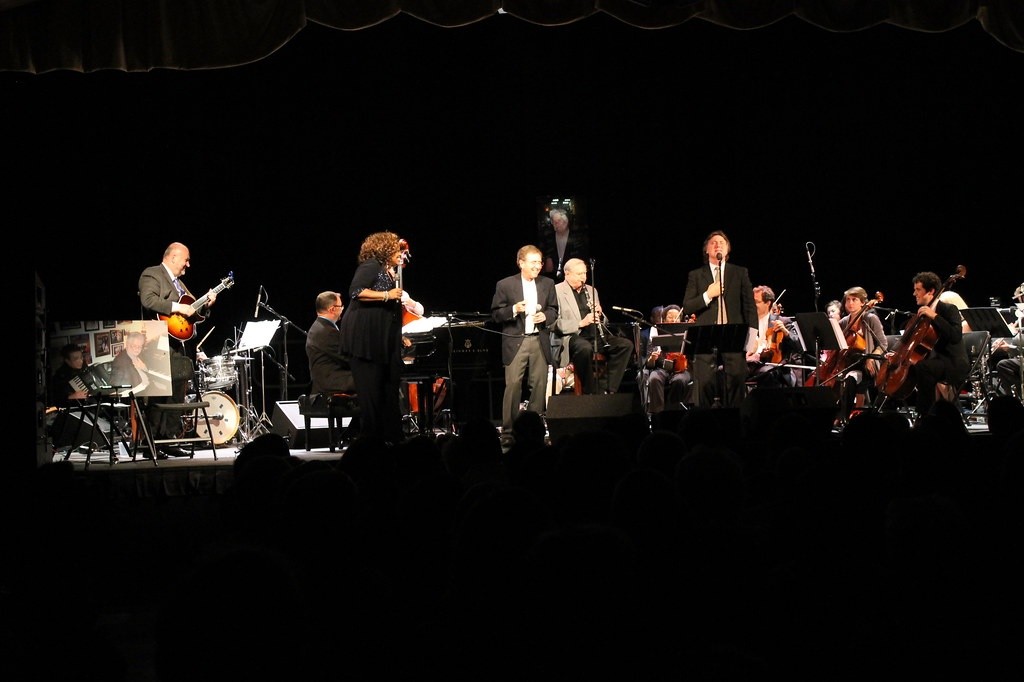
[
  {"xmin": 54, "ymin": 356, "xmax": 218, "ymax": 467},
  {"xmin": 867, "ymin": 331, "xmax": 992, "ymax": 426}
]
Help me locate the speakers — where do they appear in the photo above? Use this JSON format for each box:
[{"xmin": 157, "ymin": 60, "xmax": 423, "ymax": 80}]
[
  {"xmin": 269, "ymin": 400, "xmax": 354, "ymax": 450},
  {"xmin": 51, "ymin": 410, "xmax": 120, "ymax": 452},
  {"xmin": 544, "ymin": 392, "xmax": 647, "ymax": 444},
  {"xmin": 744, "ymin": 386, "xmax": 839, "ymax": 436}
]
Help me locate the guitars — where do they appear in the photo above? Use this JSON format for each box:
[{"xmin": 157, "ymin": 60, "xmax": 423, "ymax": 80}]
[{"xmin": 157, "ymin": 275, "xmax": 235, "ymax": 341}]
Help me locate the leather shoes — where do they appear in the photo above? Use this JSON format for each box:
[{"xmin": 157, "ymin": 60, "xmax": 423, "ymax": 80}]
[
  {"xmin": 143, "ymin": 449, "xmax": 168, "ymax": 459},
  {"xmin": 170, "ymin": 448, "xmax": 194, "ymax": 456}
]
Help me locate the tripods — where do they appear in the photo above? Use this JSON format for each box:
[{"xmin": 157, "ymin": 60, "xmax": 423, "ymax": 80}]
[{"xmin": 227, "ymin": 320, "xmax": 281, "ymax": 446}]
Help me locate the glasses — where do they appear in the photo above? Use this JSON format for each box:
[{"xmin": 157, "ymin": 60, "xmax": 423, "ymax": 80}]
[
  {"xmin": 527, "ymin": 261, "xmax": 544, "ymax": 266},
  {"xmin": 334, "ymin": 305, "xmax": 345, "ymax": 309},
  {"xmin": 756, "ymin": 300, "xmax": 764, "ymax": 304}
]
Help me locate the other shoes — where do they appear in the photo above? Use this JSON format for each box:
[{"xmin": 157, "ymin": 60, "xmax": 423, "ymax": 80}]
[{"xmin": 501, "ymin": 437, "xmax": 514, "ymax": 447}]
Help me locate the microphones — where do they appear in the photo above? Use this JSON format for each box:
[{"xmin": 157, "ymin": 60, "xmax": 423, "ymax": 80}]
[
  {"xmin": 254, "ymin": 345, "xmax": 270, "ymax": 353},
  {"xmin": 806, "ymin": 246, "xmax": 815, "ymax": 273},
  {"xmin": 255, "ymin": 285, "xmax": 263, "ymax": 317},
  {"xmin": 716, "ymin": 251, "xmax": 723, "ymax": 261},
  {"xmin": 613, "ymin": 306, "xmax": 638, "ymax": 312},
  {"xmin": 216, "ymin": 413, "xmax": 224, "ymax": 420},
  {"xmin": 534, "ymin": 304, "xmax": 542, "ymax": 330},
  {"xmin": 221, "ymin": 340, "xmax": 228, "ymax": 355}
]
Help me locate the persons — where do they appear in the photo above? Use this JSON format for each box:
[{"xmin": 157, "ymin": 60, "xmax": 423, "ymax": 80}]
[
  {"xmin": 48, "ymin": 320, "xmax": 160, "ymax": 399},
  {"xmin": 139, "ymin": 242, "xmax": 216, "ymax": 461},
  {"xmin": 683, "ymin": 229, "xmax": 758, "ymax": 409},
  {"xmin": 307, "ymin": 291, "xmax": 357, "ymax": 394},
  {"xmin": 636, "ymin": 271, "xmax": 1024, "ymax": 432},
  {"xmin": 338, "ymin": 231, "xmax": 425, "ymax": 443},
  {"xmin": 542, "ymin": 207, "xmax": 582, "ymax": 284},
  {"xmin": 548, "ymin": 257, "xmax": 634, "ymax": 395},
  {"xmin": 491, "ymin": 245, "xmax": 559, "ymax": 447},
  {"xmin": 0, "ymin": 396, "xmax": 1024, "ymax": 682}
]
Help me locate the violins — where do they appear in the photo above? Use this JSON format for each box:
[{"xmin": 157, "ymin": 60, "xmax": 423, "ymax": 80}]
[
  {"xmin": 666, "ymin": 313, "xmax": 696, "ymax": 373},
  {"xmin": 760, "ymin": 304, "xmax": 784, "ymax": 364}
]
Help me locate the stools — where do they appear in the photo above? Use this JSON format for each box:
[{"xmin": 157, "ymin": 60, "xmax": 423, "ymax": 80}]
[{"xmin": 297, "ymin": 392, "xmax": 358, "ymax": 453}]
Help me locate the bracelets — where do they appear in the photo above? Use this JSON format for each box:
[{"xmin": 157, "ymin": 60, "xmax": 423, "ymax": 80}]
[
  {"xmin": 385, "ymin": 290, "xmax": 388, "ymax": 302},
  {"xmin": 383, "ymin": 291, "xmax": 385, "ymax": 301}
]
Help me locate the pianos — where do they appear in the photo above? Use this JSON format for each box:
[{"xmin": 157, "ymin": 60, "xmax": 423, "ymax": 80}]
[
  {"xmin": 398, "ymin": 321, "xmax": 487, "ymax": 432},
  {"xmin": 68, "ymin": 362, "xmax": 116, "ymax": 398}
]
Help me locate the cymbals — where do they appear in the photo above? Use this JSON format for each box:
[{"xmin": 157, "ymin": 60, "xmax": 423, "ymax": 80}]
[{"xmin": 232, "ymin": 355, "xmax": 255, "ymax": 362}]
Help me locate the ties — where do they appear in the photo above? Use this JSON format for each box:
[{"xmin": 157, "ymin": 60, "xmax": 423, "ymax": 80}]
[{"xmin": 174, "ymin": 278, "xmax": 185, "ymax": 296}]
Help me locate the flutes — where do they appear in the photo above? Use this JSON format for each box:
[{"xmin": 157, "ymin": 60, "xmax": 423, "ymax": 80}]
[{"xmin": 582, "ymin": 280, "xmax": 612, "ymax": 350}]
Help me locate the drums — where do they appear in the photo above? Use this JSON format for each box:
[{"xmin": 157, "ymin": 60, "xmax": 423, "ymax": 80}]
[
  {"xmin": 199, "ymin": 356, "xmax": 239, "ymax": 390},
  {"xmin": 191, "ymin": 390, "xmax": 240, "ymax": 445}
]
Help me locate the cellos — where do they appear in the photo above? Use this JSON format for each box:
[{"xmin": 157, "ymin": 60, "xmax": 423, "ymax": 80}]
[
  {"xmin": 803, "ymin": 291, "xmax": 884, "ymax": 392},
  {"xmin": 877, "ymin": 265, "xmax": 966, "ymax": 412}
]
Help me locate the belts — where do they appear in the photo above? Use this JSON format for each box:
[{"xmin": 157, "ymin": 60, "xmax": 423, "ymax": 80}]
[{"xmin": 524, "ymin": 332, "xmax": 540, "ymax": 336}]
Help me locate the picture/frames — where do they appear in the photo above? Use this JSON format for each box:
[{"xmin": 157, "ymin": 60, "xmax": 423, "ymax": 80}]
[{"xmin": 45, "ymin": 320, "xmax": 125, "ymax": 364}]
